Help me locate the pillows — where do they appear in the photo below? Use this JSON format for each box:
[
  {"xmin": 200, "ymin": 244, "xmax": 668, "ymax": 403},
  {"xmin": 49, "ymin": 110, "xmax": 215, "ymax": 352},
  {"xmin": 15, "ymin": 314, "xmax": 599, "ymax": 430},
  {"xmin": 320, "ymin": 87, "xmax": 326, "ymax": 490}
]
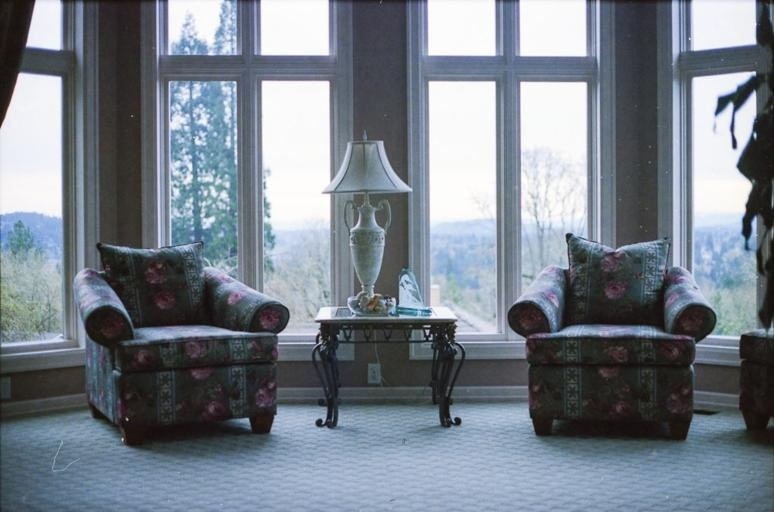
[
  {"xmin": 562, "ymin": 231, "xmax": 671, "ymax": 326},
  {"xmin": 95, "ymin": 240, "xmax": 211, "ymax": 327}
]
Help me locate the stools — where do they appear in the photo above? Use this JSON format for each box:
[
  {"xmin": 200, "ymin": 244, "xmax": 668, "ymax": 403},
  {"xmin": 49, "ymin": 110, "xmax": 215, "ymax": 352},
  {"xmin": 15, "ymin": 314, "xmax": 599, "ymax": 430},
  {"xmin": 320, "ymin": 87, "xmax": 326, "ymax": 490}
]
[{"xmin": 738, "ymin": 328, "xmax": 774, "ymax": 433}]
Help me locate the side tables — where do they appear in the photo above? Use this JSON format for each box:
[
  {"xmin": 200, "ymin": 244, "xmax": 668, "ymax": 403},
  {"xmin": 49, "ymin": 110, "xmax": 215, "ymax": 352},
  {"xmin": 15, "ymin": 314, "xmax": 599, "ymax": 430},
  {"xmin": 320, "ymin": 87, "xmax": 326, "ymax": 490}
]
[{"xmin": 311, "ymin": 305, "xmax": 465, "ymax": 428}]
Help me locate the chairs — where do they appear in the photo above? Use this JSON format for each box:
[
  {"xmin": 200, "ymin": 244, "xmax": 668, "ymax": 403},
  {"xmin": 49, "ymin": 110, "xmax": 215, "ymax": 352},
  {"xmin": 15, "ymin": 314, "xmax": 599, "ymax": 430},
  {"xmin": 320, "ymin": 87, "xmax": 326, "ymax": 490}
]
[
  {"xmin": 507, "ymin": 264, "xmax": 717, "ymax": 440},
  {"xmin": 72, "ymin": 257, "xmax": 290, "ymax": 445}
]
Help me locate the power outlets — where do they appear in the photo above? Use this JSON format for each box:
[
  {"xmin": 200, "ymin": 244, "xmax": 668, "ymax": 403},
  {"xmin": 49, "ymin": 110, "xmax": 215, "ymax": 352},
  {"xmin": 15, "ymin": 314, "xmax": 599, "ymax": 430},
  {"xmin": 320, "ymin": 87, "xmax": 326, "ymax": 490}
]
[{"xmin": 366, "ymin": 363, "xmax": 382, "ymax": 384}]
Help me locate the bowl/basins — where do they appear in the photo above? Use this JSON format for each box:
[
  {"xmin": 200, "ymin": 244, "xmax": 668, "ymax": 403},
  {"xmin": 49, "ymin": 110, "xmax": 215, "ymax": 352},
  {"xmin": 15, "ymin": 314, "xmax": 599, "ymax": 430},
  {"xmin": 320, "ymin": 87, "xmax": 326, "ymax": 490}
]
[{"xmin": 347, "ymin": 296, "xmax": 396, "ymax": 316}]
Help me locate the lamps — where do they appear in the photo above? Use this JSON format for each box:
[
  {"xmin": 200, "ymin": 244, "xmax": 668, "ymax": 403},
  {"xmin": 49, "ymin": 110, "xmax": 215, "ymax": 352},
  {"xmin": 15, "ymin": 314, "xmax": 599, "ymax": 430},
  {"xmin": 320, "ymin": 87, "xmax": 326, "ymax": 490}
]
[{"xmin": 321, "ymin": 129, "xmax": 413, "ymax": 293}]
